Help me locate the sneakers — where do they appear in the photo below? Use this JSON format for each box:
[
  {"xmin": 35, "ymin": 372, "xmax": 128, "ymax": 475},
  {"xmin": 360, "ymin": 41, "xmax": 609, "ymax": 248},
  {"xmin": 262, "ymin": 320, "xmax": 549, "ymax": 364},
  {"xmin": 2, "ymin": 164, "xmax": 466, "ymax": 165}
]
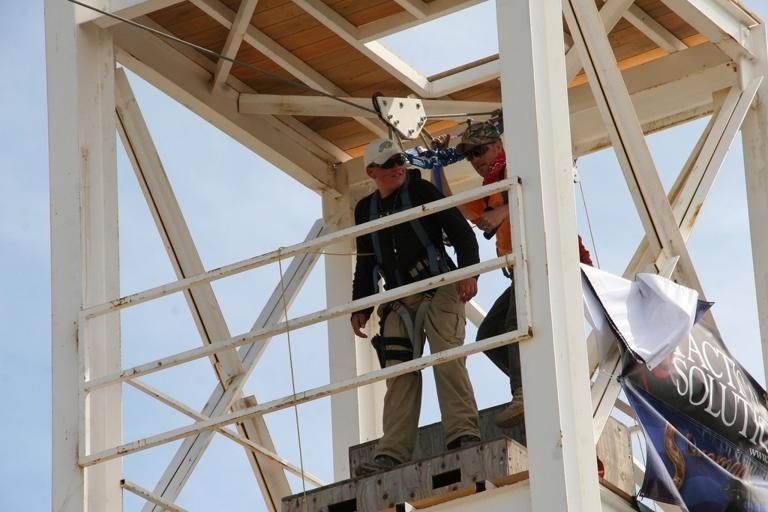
[
  {"xmin": 447, "ymin": 435, "xmax": 480, "ymax": 450},
  {"xmin": 495, "ymin": 385, "xmax": 524, "ymax": 428},
  {"xmin": 356, "ymin": 456, "xmax": 401, "ymax": 476}
]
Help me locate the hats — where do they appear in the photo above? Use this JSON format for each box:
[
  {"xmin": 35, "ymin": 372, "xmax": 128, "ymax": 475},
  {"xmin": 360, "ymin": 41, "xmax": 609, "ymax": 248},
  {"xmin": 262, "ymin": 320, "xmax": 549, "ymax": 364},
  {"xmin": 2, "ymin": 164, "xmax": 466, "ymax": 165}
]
[
  {"xmin": 364, "ymin": 139, "xmax": 402, "ymax": 166},
  {"xmin": 455, "ymin": 123, "xmax": 501, "ymax": 150}
]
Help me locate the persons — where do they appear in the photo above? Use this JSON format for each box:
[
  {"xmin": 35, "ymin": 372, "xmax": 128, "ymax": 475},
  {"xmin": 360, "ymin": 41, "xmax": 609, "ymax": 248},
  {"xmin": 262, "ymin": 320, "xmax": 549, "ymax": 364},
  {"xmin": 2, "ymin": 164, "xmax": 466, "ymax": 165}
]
[
  {"xmin": 430, "ymin": 122, "xmax": 594, "ymax": 427},
  {"xmin": 350, "ymin": 138, "xmax": 480, "ymax": 474}
]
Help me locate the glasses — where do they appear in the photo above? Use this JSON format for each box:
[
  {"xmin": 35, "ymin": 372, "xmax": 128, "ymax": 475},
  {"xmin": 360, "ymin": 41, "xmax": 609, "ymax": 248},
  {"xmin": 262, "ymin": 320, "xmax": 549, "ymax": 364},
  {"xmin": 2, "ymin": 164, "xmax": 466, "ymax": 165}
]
[
  {"xmin": 371, "ymin": 155, "xmax": 405, "ymax": 169},
  {"xmin": 463, "ymin": 142, "xmax": 496, "ymax": 161}
]
